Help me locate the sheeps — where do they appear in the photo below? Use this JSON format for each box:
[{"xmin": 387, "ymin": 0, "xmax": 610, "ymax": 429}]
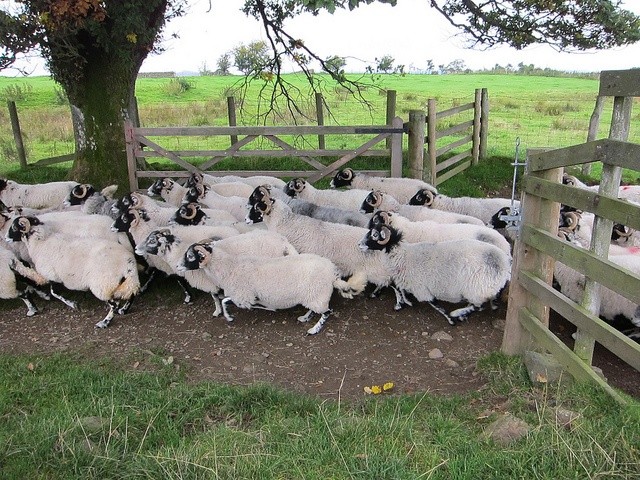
[
  {"xmin": 146, "ymin": 178, "xmax": 254, "ymax": 205},
  {"xmin": 135, "ymin": 229, "xmax": 299, "ymax": 318},
  {"xmin": 6, "ymin": 216, "xmax": 141, "ymax": 329},
  {"xmin": 244, "ymin": 194, "xmax": 398, "ymax": 299},
  {"xmin": 0, "ymin": 200, "xmax": 66, "ymax": 213},
  {"xmin": 111, "ymin": 209, "xmax": 158, "ymax": 245},
  {"xmin": 181, "ymin": 178, "xmax": 251, "ymax": 210},
  {"xmin": 368, "ymin": 211, "xmax": 512, "ymax": 257},
  {"xmin": 411, "ymin": 185, "xmax": 520, "ymax": 220},
  {"xmin": 359, "ymin": 189, "xmax": 484, "ymax": 224},
  {"xmin": 0, "ymin": 238, "xmax": 82, "ymax": 319},
  {"xmin": 358, "ymin": 223, "xmax": 507, "ymax": 325},
  {"xmin": 490, "ymin": 206, "xmax": 519, "ymax": 233},
  {"xmin": 110, "ymin": 192, "xmax": 177, "ymax": 226},
  {"xmin": 283, "ymin": 177, "xmax": 369, "ymax": 210},
  {"xmin": 168, "ymin": 202, "xmax": 238, "ymax": 225},
  {"xmin": 247, "ymin": 185, "xmax": 370, "ymax": 229},
  {"xmin": 188, "ymin": 172, "xmax": 286, "ymax": 191},
  {"xmin": 329, "ymin": 167, "xmax": 439, "ymax": 205},
  {"xmin": 177, "ymin": 243, "xmax": 368, "ymax": 336},
  {"xmin": 549, "ymin": 173, "xmax": 640, "ymax": 331},
  {"xmin": 0, "ymin": 178, "xmax": 80, "ymax": 209},
  {"xmin": 63, "ymin": 183, "xmax": 118, "ymax": 214},
  {"xmin": 39, "ymin": 212, "xmax": 132, "ymax": 252},
  {"xmin": 0, "ymin": 215, "xmax": 14, "ymax": 237}
]
